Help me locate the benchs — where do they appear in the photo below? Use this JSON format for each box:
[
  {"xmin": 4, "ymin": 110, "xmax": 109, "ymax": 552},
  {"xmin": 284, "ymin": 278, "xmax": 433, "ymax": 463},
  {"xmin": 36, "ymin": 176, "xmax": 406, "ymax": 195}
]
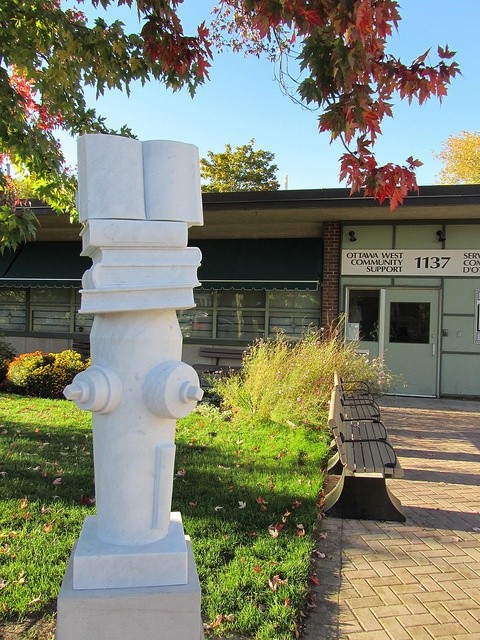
[
  {"xmin": 331, "ymin": 371, "xmax": 385, "ymax": 418},
  {"xmin": 191, "ymin": 345, "xmax": 254, "ymax": 396},
  {"xmin": 319, "ymin": 388, "xmax": 407, "ymax": 527}
]
